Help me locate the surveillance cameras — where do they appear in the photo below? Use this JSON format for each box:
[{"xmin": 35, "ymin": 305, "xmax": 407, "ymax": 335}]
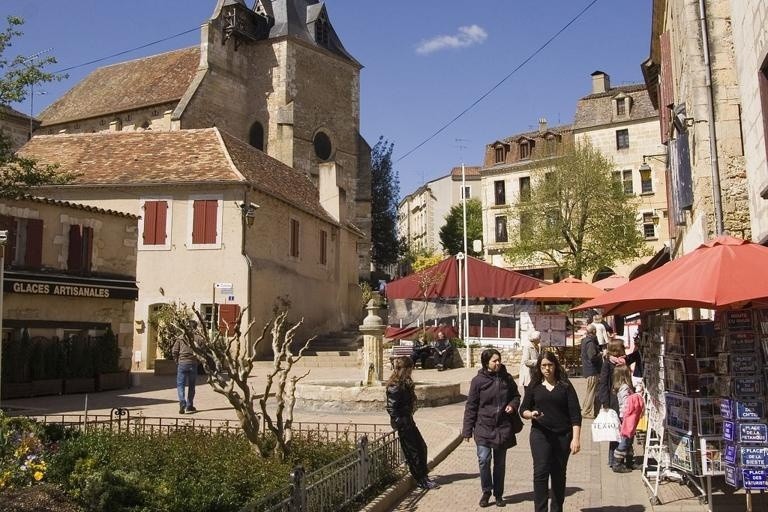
[
  {"xmin": 238, "ymin": 200, "xmax": 247, "ymax": 209},
  {"xmin": 250, "ymin": 201, "xmax": 261, "ymax": 211}
]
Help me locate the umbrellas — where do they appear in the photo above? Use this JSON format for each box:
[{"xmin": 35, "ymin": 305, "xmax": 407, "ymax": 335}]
[
  {"xmin": 570, "ymin": 235, "xmax": 768, "ymax": 319},
  {"xmin": 596, "ymin": 274, "xmax": 630, "ymax": 288},
  {"xmin": 510, "ymin": 275, "xmax": 610, "ymax": 348}
]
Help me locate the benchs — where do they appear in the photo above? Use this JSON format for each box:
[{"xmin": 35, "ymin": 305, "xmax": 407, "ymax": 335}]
[{"xmin": 389, "ymin": 345, "xmax": 454, "ymax": 369}]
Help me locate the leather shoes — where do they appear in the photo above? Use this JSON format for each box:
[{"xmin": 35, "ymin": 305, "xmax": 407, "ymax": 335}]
[
  {"xmin": 479, "ymin": 492, "xmax": 492, "ymax": 507},
  {"xmin": 495, "ymin": 496, "xmax": 506, "ymax": 507}
]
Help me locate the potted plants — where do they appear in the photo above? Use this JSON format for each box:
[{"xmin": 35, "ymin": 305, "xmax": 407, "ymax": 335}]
[{"xmin": 1, "ymin": 324, "xmax": 131, "ymax": 400}]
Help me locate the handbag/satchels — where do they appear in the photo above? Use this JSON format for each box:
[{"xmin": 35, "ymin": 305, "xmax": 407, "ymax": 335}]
[
  {"xmin": 510, "ymin": 413, "xmax": 523, "ymax": 433},
  {"xmin": 591, "ymin": 408, "xmax": 623, "ymax": 441}
]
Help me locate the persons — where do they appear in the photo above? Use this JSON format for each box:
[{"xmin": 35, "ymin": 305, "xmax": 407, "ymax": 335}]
[
  {"xmin": 462, "ymin": 349, "xmax": 520, "ymax": 507},
  {"xmin": 412, "ymin": 334, "xmax": 431, "ymax": 367},
  {"xmin": 434, "ymin": 331, "xmax": 452, "ymax": 371},
  {"xmin": 582, "ymin": 312, "xmax": 648, "ymax": 472},
  {"xmin": 386, "ymin": 356, "xmax": 438, "ymax": 489},
  {"xmin": 522, "ymin": 331, "xmax": 542, "ymax": 392},
  {"xmin": 171, "ymin": 320, "xmax": 205, "ymax": 413},
  {"xmin": 523, "ymin": 352, "xmax": 581, "ymax": 511}
]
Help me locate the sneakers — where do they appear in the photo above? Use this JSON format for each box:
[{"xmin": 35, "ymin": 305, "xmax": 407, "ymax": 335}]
[{"xmin": 419, "ymin": 477, "xmax": 440, "ymax": 489}]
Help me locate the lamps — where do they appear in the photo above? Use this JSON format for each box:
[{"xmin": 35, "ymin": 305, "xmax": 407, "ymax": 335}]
[
  {"xmin": 672, "ymin": 113, "xmax": 694, "ymax": 134},
  {"xmin": 639, "ymin": 153, "xmax": 669, "ymax": 182},
  {"xmin": 242, "ymin": 207, "xmax": 255, "ymax": 229},
  {"xmin": 651, "ymin": 208, "xmax": 669, "ymax": 226}
]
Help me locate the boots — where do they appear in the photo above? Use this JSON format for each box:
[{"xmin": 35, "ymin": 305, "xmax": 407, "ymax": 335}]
[
  {"xmin": 624, "ymin": 451, "xmax": 643, "ymax": 470},
  {"xmin": 612, "ymin": 447, "xmax": 632, "ymax": 473}
]
